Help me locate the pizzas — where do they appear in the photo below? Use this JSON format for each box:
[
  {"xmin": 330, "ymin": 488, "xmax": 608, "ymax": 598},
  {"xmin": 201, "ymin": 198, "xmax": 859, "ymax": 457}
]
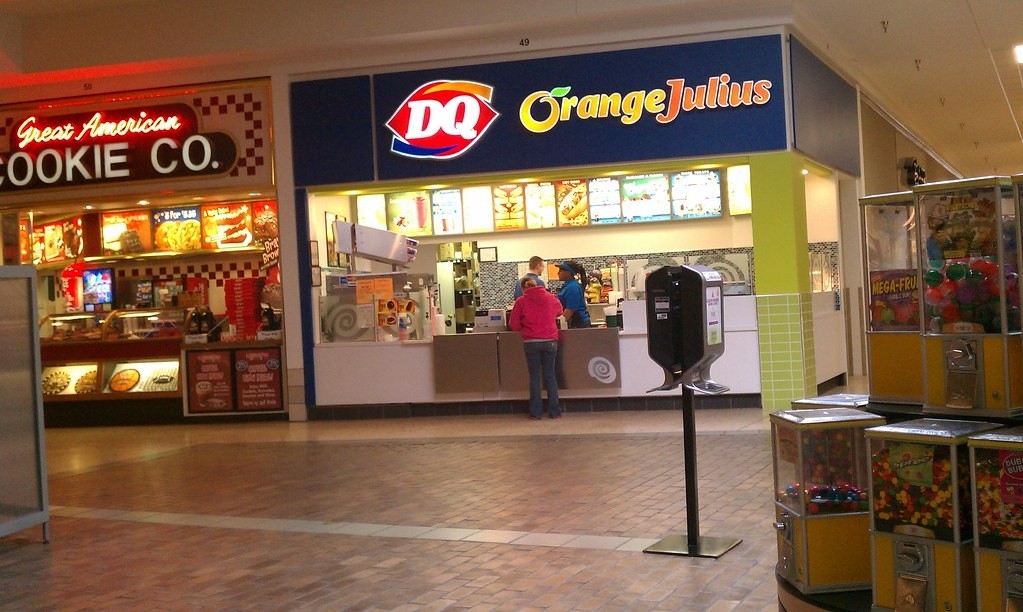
[
  {"xmin": 155, "ymin": 219, "xmax": 201, "ymax": 251},
  {"xmin": 109, "ymin": 369, "xmax": 140, "ymax": 391},
  {"xmin": 74, "ymin": 370, "xmax": 97, "ymax": 394},
  {"xmin": 41, "ymin": 371, "xmax": 70, "ymax": 394}
]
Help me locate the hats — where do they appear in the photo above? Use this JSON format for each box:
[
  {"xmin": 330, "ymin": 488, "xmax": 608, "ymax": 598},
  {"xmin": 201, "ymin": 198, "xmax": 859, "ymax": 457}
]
[{"xmin": 554, "ymin": 261, "xmax": 576, "ymax": 275}]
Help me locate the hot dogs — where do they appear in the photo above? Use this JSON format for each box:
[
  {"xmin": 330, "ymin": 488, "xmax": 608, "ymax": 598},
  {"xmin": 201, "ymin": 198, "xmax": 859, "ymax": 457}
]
[{"xmin": 558, "ymin": 183, "xmax": 588, "ymax": 220}]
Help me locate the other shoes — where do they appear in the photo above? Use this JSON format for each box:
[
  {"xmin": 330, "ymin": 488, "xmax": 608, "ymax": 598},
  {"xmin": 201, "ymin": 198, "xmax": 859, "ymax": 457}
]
[
  {"xmin": 530, "ymin": 415, "xmax": 540, "ymax": 420},
  {"xmin": 548, "ymin": 414, "xmax": 561, "ymax": 419}
]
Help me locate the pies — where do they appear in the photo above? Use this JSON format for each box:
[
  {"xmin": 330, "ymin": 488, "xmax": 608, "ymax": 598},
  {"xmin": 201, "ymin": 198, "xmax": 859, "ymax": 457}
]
[{"xmin": 493, "ymin": 186, "xmax": 524, "ymax": 214}]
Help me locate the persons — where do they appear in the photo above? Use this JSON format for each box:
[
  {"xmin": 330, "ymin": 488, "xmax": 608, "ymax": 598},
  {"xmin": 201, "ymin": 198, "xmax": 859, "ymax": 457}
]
[
  {"xmin": 553, "ymin": 260, "xmax": 591, "ymax": 328},
  {"xmin": 514, "ymin": 256, "xmax": 546, "ymax": 304},
  {"xmin": 509, "ymin": 277, "xmax": 563, "ymax": 420}
]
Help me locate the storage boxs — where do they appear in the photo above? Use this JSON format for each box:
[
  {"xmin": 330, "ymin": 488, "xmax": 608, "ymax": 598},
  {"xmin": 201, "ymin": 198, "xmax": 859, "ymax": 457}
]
[
  {"xmin": 258, "ymin": 330, "xmax": 282, "ymax": 345},
  {"xmin": 185, "ymin": 334, "xmax": 212, "ymax": 343},
  {"xmin": 224, "ymin": 276, "xmax": 266, "ymax": 336}
]
